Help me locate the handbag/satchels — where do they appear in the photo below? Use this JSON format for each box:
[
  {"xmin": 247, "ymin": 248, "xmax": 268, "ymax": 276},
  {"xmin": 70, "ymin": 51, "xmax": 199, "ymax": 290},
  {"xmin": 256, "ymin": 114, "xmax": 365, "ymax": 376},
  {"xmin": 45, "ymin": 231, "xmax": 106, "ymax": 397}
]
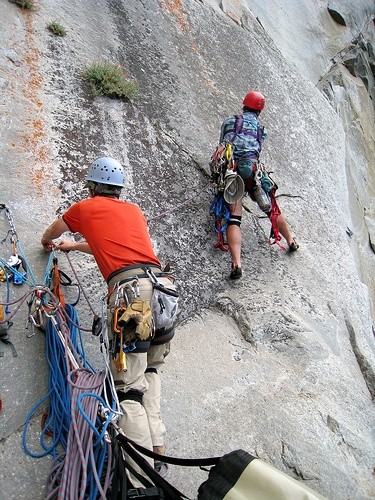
[
  {"xmin": 150, "ymin": 282, "xmax": 180, "ymax": 329},
  {"xmin": 260, "ymin": 170, "xmax": 275, "ymax": 193},
  {"xmin": 236, "ymin": 159, "xmax": 252, "ymax": 179}
]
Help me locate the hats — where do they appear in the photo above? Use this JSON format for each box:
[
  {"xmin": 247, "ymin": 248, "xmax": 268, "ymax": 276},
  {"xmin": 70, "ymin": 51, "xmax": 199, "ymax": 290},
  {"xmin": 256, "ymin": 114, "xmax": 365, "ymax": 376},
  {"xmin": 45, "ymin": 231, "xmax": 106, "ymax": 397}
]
[{"xmin": 223, "ymin": 169, "xmax": 245, "ymax": 204}]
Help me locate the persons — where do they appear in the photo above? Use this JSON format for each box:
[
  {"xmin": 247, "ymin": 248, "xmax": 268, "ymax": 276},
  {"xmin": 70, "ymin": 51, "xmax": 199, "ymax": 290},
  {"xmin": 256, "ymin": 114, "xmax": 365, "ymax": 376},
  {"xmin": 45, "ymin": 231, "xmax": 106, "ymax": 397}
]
[
  {"xmin": 219, "ymin": 91, "xmax": 299, "ymax": 279},
  {"xmin": 41, "ymin": 156, "xmax": 178, "ymax": 499}
]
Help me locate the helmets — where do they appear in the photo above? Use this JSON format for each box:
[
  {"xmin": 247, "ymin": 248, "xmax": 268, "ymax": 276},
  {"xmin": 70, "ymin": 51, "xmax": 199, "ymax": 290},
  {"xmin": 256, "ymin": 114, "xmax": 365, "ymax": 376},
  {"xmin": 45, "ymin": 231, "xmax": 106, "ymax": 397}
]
[
  {"xmin": 242, "ymin": 91, "xmax": 265, "ymax": 110},
  {"xmin": 85, "ymin": 156, "xmax": 126, "ymax": 188}
]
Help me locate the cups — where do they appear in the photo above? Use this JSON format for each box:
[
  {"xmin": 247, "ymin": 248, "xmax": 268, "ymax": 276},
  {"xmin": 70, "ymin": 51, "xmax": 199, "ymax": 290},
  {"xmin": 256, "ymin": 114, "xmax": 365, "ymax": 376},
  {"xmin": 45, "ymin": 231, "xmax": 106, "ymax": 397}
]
[{"xmin": 253, "ymin": 186, "xmax": 271, "ymax": 211}]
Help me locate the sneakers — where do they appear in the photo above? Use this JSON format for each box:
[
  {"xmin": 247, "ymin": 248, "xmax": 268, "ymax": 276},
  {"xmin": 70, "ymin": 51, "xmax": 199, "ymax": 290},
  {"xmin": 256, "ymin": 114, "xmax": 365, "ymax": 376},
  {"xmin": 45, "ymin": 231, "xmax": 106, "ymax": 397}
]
[
  {"xmin": 154, "ymin": 460, "xmax": 169, "ymax": 479},
  {"xmin": 229, "ymin": 265, "xmax": 243, "ymax": 278},
  {"xmin": 288, "ymin": 239, "xmax": 299, "ymax": 251}
]
